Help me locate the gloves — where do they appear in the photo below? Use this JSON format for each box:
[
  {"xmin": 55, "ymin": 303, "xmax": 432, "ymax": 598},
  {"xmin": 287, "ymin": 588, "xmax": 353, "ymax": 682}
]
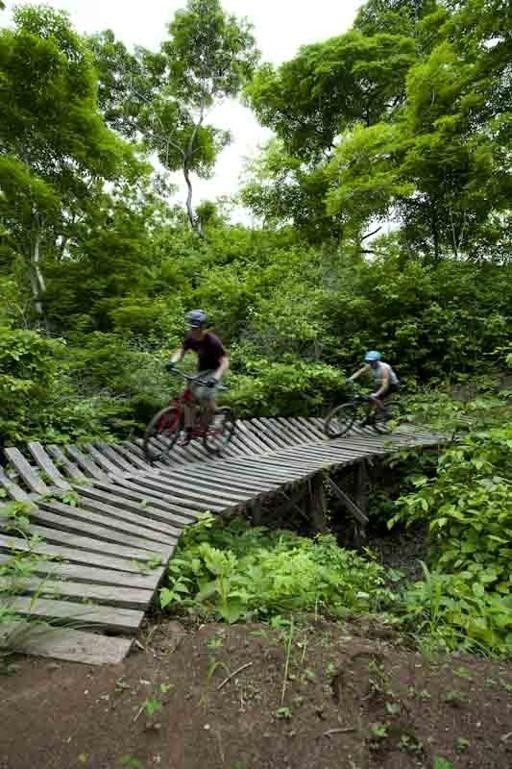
[
  {"xmin": 205, "ymin": 378, "xmax": 217, "ymax": 388},
  {"xmin": 165, "ymin": 361, "xmax": 174, "ymax": 371}
]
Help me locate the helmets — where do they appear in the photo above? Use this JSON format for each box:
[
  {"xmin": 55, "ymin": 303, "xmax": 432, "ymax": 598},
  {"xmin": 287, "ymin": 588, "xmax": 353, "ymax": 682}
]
[
  {"xmin": 364, "ymin": 351, "xmax": 381, "ymax": 363},
  {"xmin": 184, "ymin": 309, "xmax": 208, "ymax": 328}
]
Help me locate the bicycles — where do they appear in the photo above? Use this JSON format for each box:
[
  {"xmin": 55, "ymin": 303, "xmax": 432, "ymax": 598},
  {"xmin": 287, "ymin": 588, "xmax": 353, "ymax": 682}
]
[
  {"xmin": 322, "ymin": 380, "xmax": 405, "ymax": 439},
  {"xmin": 142, "ymin": 365, "xmax": 238, "ymax": 461}
]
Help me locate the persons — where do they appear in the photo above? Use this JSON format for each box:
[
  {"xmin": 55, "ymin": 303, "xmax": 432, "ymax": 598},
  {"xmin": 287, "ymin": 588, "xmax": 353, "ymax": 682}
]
[
  {"xmin": 345, "ymin": 351, "xmax": 400, "ymax": 428},
  {"xmin": 164, "ymin": 309, "xmax": 228, "ymax": 446}
]
